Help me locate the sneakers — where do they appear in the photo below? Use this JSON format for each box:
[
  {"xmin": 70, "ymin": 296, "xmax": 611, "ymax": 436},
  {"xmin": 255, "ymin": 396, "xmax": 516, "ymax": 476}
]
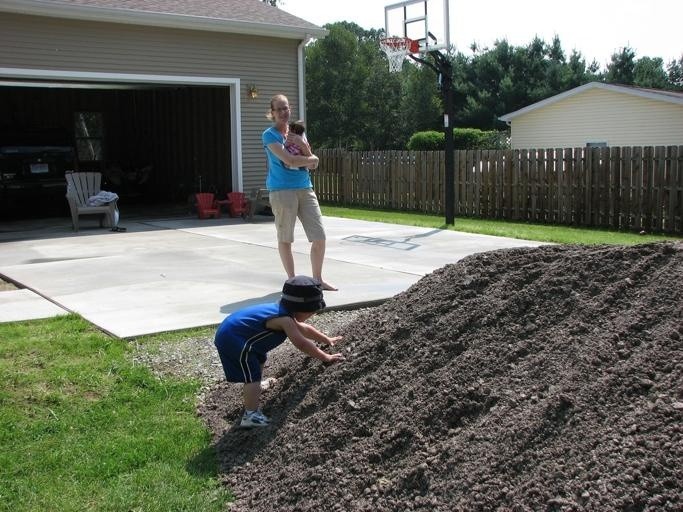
[{"xmin": 240, "ymin": 410, "xmax": 272, "ymax": 428}]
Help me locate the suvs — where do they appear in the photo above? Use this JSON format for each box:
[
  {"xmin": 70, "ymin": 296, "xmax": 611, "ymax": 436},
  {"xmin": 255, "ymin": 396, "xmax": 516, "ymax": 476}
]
[{"xmin": 0, "ymin": 140, "xmax": 79, "ymax": 220}]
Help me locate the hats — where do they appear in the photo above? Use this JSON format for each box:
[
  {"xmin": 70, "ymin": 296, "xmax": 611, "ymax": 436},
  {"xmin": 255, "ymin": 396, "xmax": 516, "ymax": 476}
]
[{"xmin": 280, "ymin": 276, "xmax": 326, "ymax": 313}]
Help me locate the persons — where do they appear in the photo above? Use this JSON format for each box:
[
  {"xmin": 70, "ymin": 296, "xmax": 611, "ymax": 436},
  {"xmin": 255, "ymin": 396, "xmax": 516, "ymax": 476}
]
[
  {"xmin": 261, "ymin": 93, "xmax": 339, "ymax": 291},
  {"xmin": 283, "ymin": 119, "xmax": 312, "ymax": 171},
  {"xmin": 213, "ymin": 274, "xmax": 346, "ymax": 427}
]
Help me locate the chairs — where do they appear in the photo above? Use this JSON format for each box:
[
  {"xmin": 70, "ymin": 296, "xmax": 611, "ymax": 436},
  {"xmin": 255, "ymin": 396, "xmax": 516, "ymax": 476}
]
[
  {"xmin": 194, "ymin": 193, "xmax": 221, "ymax": 219},
  {"xmin": 64, "ymin": 171, "xmax": 120, "ymax": 232},
  {"xmin": 227, "ymin": 192, "xmax": 252, "ymax": 217}
]
